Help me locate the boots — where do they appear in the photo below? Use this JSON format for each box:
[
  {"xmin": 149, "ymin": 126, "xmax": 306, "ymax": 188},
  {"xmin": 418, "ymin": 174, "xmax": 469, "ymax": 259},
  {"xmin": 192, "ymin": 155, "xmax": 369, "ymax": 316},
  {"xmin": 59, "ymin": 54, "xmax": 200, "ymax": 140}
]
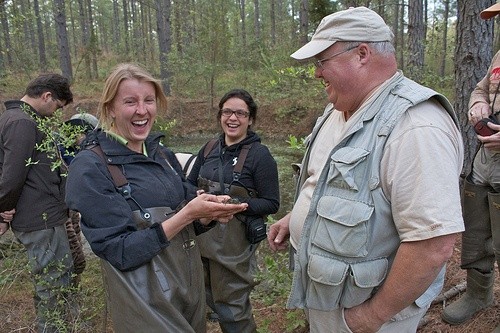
[{"xmin": 441, "ymin": 265, "xmax": 494, "ymax": 325}]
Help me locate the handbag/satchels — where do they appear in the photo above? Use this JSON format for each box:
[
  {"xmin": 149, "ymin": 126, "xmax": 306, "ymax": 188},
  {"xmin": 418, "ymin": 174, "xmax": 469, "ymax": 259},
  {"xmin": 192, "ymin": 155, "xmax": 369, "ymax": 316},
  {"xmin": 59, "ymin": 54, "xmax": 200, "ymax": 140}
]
[{"xmin": 244, "ymin": 215, "xmax": 267, "ymax": 243}]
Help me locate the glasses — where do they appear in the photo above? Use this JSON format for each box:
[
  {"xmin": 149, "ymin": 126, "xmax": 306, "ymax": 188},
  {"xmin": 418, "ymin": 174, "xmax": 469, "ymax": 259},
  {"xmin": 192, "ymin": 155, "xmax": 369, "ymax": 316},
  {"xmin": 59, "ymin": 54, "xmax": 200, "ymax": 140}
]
[
  {"xmin": 220, "ymin": 107, "xmax": 251, "ymax": 119},
  {"xmin": 313, "ymin": 46, "xmax": 359, "ymax": 68}
]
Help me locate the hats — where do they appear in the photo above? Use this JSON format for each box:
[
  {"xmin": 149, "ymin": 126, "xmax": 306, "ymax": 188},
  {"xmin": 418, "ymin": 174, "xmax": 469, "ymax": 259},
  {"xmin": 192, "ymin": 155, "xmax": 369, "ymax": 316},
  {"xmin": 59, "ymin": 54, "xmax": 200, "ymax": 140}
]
[
  {"xmin": 480, "ymin": 1, "xmax": 500, "ymax": 19},
  {"xmin": 290, "ymin": 6, "xmax": 395, "ymax": 59}
]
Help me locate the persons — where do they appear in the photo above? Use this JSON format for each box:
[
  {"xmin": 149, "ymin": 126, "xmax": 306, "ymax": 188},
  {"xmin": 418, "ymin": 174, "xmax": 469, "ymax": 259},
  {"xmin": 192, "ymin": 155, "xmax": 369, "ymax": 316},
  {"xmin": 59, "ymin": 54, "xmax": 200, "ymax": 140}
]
[
  {"xmin": 0, "ymin": 73, "xmax": 87, "ymax": 333},
  {"xmin": 41, "ymin": 113, "xmax": 86, "ymax": 321},
  {"xmin": 186, "ymin": 87, "xmax": 281, "ymax": 333},
  {"xmin": 57, "ymin": 112, "xmax": 99, "ymax": 164},
  {"xmin": 267, "ymin": 6, "xmax": 465, "ymax": 333},
  {"xmin": 439, "ymin": 1, "xmax": 500, "ymax": 327},
  {"xmin": 66, "ymin": 62, "xmax": 248, "ymax": 333}
]
